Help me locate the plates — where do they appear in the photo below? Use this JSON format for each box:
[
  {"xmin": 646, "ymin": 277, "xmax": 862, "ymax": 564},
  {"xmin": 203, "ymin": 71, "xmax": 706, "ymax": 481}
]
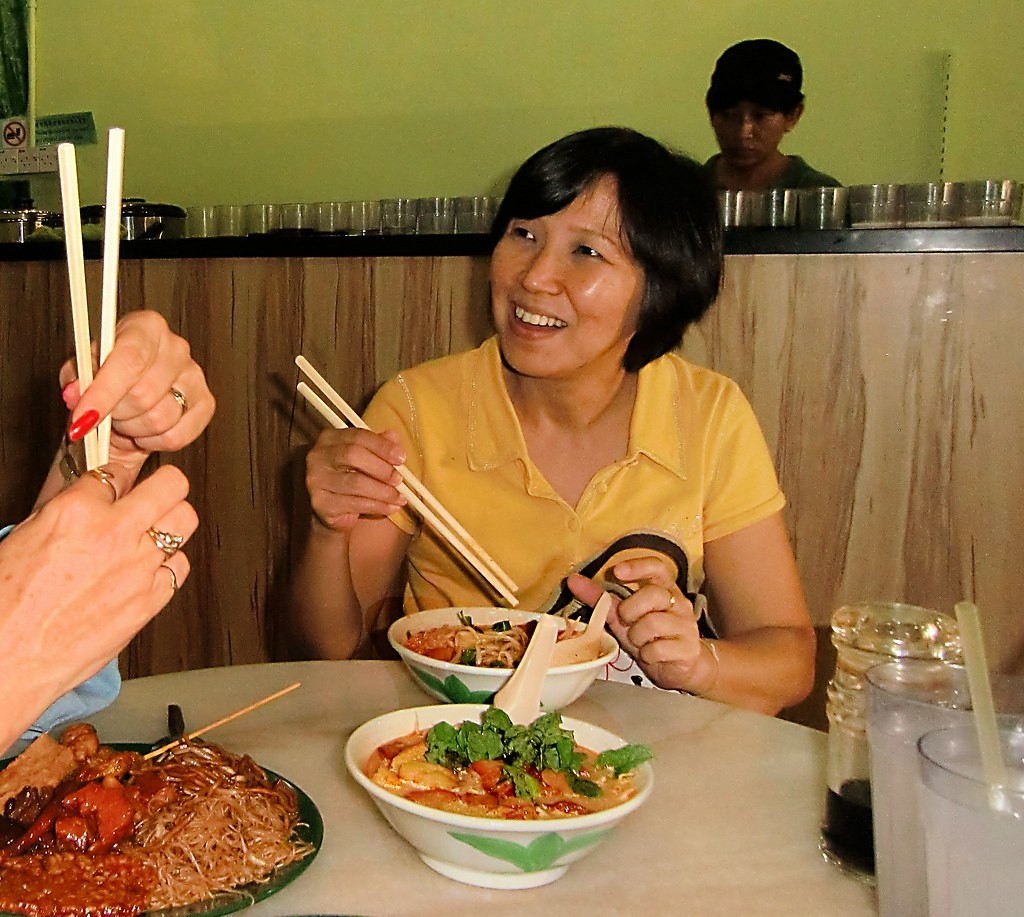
[{"xmin": 1, "ymin": 742, "xmax": 323, "ymax": 915}]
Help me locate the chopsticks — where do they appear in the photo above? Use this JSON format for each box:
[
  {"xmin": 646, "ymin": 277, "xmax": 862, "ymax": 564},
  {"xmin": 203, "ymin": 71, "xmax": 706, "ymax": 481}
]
[
  {"xmin": 296, "ymin": 355, "xmax": 519, "ymax": 607},
  {"xmin": 56, "ymin": 127, "xmax": 127, "ymax": 472}
]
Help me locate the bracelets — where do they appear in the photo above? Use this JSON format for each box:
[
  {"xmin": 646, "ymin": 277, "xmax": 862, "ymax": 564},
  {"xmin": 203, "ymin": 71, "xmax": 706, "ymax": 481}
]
[{"xmin": 689, "ymin": 637, "xmax": 721, "ymax": 697}]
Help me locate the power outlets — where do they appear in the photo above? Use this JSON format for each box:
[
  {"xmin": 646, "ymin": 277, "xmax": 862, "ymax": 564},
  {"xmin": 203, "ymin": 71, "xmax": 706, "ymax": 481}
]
[{"xmin": 0, "ymin": 147, "xmax": 59, "ymax": 175}]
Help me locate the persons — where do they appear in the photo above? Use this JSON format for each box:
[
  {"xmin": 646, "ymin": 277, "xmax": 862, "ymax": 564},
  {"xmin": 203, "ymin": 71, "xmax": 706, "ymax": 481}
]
[
  {"xmin": 700, "ymin": 38, "xmax": 848, "ymax": 225},
  {"xmin": 285, "ymin": 125, "xmax": 820, "ymax": 721},
  {"xmin": 0, "ymin": 309, "xmax": 216, "ymax": 768}
]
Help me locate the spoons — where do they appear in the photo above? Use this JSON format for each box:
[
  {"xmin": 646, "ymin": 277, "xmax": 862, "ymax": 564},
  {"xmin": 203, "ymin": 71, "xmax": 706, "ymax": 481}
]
[
  {"xmin": 493, "ymin": 613, "xmax": 558, "ymax": 726},
  {"xmin": 549, "ymin": 589, "xmax": 613, "ymax": 668}
]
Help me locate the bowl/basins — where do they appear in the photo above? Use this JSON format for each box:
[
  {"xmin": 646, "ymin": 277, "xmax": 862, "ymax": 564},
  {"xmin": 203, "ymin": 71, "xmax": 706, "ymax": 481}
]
[
  {"xmin": 344, "ymin": 704, "xmax": 654, "ymax": 890},
  {"xmin": 387, "ymin": 608, "xmax": 619, "ymax": 712}
]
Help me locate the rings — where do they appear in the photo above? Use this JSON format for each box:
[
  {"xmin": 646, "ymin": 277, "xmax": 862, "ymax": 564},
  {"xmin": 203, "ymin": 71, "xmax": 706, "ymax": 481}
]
[
  {"xmin": 146, "ymin": 526, "xmax": 184, "ymax": 563},
  {"xmin": 666, "ymin": 588, "xmax": 676, "ymax": 611},
  {"xmin": 169, "ymin": 386, "xmax": 189, "ymax": 417},
  {"xmin": 161, "ymin": 566, "xmax": 179, "ymax": 594},
  {"xmin": 84, "ymin": 466, "xmax": 117, "ymax": 504}
]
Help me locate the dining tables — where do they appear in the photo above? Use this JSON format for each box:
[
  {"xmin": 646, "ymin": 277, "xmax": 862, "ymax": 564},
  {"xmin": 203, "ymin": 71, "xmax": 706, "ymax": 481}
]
[{"xmin": 0, "ymin": 660, "xmax": 880, "ymax": 917}]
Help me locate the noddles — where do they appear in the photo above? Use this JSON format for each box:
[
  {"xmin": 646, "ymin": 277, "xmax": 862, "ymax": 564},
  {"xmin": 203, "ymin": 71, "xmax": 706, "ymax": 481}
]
[{"xmin": 457, "ymin": 625, "xmax": 529, "ymax": 668}]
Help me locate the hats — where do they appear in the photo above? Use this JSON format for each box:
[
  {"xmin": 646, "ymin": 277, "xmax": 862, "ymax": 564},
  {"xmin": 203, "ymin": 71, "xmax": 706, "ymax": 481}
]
[{"xmin": 710, "ymin": 37, "xmax": 805, "ymax": 116}]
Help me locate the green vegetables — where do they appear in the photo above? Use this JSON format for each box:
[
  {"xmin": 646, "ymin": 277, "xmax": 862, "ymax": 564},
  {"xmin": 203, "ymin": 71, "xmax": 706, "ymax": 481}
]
[
  {"xmin": 459, "ymin": 610, "xmax": 511, "ymax": 669},
  {"xmin": 424, "ymin": 707, "xmax": 660, "ymax": 802}
]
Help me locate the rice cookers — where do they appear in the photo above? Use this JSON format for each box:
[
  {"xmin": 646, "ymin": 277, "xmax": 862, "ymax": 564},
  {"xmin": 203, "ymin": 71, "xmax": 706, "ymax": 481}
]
[{"xmin": 58, "ymin": 197, "xmax": 188, "ymax": 240}]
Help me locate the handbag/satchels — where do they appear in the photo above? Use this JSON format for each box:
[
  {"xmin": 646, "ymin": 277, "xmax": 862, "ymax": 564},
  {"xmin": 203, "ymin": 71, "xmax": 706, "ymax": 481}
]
[{"xmin": 541, "ymin": 534, "xmax": 718, "ymax": 697}]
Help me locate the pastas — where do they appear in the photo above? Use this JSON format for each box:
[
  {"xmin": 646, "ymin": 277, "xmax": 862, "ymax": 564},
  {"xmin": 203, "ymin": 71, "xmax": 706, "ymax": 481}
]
[{"xmin": 129, "ymin": 733, "xmax": 298, "ymax": 851}]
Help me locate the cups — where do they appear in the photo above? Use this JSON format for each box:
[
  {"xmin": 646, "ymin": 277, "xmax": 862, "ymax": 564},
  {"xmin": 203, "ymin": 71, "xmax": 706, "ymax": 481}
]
[
  {"xmin": 863, "ymin": 660, "xmax": 1023, "ymax": 917},
  {"xmin": 187, "ymin": 195, "xmax": 502, "ymax": 237},
  {"xmin": 714, "ymin": 180, "xmax": 1024, "ymax": 230}
]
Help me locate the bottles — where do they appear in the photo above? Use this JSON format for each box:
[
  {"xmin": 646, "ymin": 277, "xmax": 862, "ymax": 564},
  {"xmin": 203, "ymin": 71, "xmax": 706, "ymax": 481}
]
[{"xmin": 818, "ymin": 601, "xmax": 963, "ymax": 886}]
